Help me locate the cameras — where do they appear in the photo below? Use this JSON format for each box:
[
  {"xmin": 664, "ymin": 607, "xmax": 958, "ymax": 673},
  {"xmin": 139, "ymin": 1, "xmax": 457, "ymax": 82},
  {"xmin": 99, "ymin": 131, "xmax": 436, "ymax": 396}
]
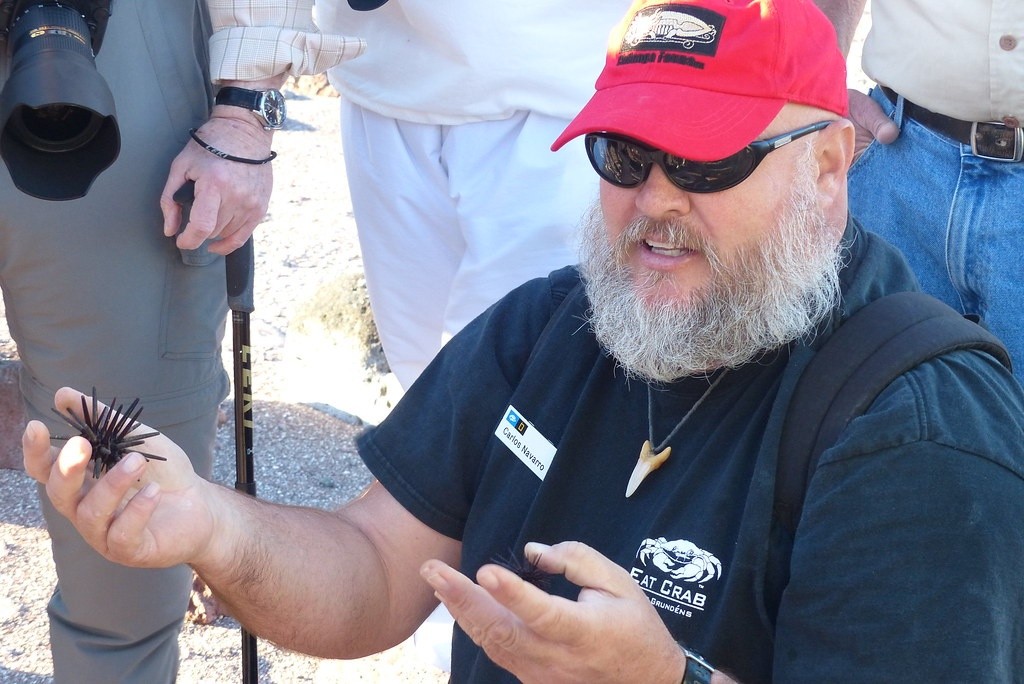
[{"xmin": 0, "ymin": 0, "xmax": 122, "ymax": 201}]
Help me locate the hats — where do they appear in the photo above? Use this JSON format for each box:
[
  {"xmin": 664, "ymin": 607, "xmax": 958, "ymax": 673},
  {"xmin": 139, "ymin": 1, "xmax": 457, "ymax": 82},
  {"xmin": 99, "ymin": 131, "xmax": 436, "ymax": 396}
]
[{"xmin": 551, "ymin": 0, "xmax": 849, "ymax": 152}]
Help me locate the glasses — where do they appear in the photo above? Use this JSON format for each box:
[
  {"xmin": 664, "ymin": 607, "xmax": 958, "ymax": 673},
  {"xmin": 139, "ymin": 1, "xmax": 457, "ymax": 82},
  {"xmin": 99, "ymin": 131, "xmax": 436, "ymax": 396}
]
[{"xmin": 585, "ymin": 120, "xmax": 837, "ymax": 192}]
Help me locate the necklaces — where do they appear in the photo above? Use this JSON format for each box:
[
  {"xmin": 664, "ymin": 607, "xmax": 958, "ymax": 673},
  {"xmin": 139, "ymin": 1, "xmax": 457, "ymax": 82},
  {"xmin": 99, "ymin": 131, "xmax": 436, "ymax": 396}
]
[{"xmin": 624, "ymin": 364, "xmax": 731, "ymax": 500}]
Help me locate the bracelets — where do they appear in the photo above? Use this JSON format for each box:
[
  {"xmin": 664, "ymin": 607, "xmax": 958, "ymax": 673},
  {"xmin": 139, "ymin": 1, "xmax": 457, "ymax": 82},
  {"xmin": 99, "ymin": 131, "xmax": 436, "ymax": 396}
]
[{"xmin": 187, "ymin": 126, "xmax": 278, "ymax": 166}]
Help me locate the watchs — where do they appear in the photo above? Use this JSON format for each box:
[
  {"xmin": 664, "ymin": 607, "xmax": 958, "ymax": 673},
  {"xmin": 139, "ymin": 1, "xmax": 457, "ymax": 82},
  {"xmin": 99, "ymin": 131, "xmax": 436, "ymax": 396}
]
[
  {"xmin": 673, "ymin": 638, "xmax": 715, "ymax": 684},
  {"xmin": 213, "ymin": 85, "xmax": 288, "ymax": 132}
]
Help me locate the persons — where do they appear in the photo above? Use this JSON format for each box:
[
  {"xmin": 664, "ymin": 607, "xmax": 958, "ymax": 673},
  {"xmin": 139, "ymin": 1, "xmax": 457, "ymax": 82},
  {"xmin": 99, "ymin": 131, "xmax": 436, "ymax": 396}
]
[
  {"xmin": 1, "ymin": 0, "xmax": 370, "ymax": 684},
  {"xmin": 21, "ymin": 0, "xmax": 1024, "ymax": 684}
]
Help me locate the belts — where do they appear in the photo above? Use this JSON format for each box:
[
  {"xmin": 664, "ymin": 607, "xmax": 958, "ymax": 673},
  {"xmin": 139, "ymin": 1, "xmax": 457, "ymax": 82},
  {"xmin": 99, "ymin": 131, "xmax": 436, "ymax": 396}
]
[{"xmin": 880, "ymin": 84, "xmax": 1024, "ymax": 162}]
